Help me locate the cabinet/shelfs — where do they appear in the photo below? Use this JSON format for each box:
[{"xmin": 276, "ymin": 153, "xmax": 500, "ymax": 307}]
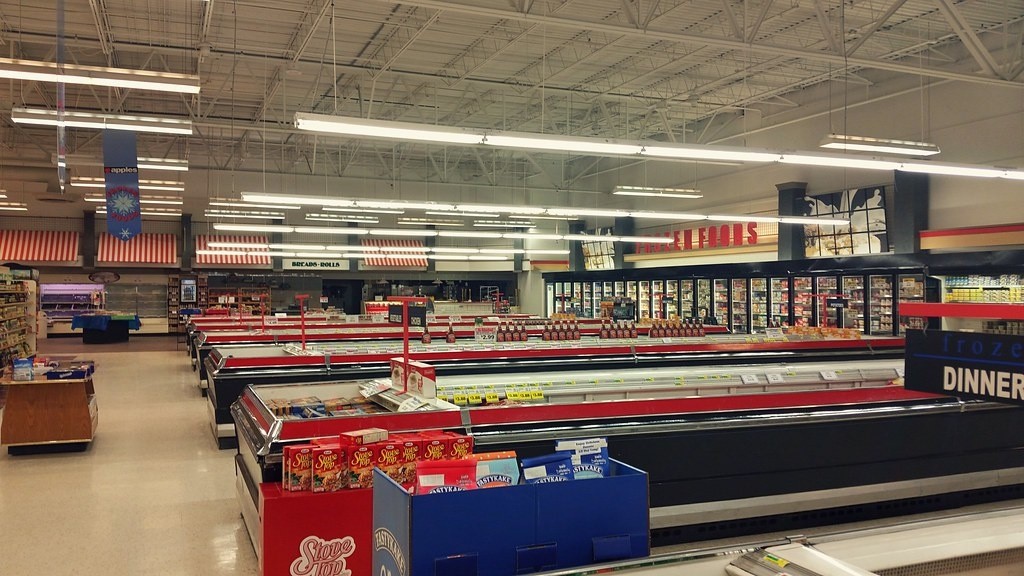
[{"xmin": 0, "ymin": 279, "xmax": 37, "ymax": 372}]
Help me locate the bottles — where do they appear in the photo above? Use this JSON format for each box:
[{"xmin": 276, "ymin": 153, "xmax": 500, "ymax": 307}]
[
  {"xmin": 446, "ymin": 322, "xmax": 455, "ymax": 343},
  {"xmin": 542, "ymin": 321, "xmax": 581, "ymax": 340},
  {"xmin": 650, "ymin": 319, "xmax": 705, "ymax": 338},
  {"xmin": 599, "ymin": 319, "xmax": 638, "ymax": 338},
  {"xmin": 422, "ymin": 323, "xmax": 431, "ymax": 343},
  {"xmin": 496, "ymin": 322, "xmax": 528, "ymax": 342}
]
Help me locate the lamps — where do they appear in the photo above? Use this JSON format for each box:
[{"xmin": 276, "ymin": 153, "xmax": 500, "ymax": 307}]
[
  {"xmin": 432, "ymin": 277, "xmax": 443, "ymax": 284},
  {"xmin": 95, "ymin": 188, "xmax": 183, "ymax": 216},
  {"xmin": 10, "ymin": 79, "xmax": 193, "ymax": 135},
  {"xmin": 0, "ymin": 181, "xmax": 28, "ymax": 211},
  {"xmin": 70, "ymin": 132, "xmax": 184, "ymax": 192},
  {"xmin": 83, "ymin": 165, "xmax": 184, "ymax": 205},
  {"xmin": 377, "ymin": 274, "xmax": 389, "ymax": 284},
  {"xmin": 0, "ymin": 147, "xmax": 8, "ymax": 199},
  {"xmin": 196, "ymin": 0, "xmax": 1024, "ymax": 261},
  {"xmin": 0, "ymin": 0, "xmax": 201, "ymax": 95},
  {"xmin": 51, "ymin": 128, "xmax": 189, "ymax": 172}
]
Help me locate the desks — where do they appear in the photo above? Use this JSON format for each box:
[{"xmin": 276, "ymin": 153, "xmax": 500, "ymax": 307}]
[
  {"xmin": 0, "ymin": 369, "xmax": 99, "ymax": 457},
  {"xmin": 71, "ymin": 315, "xmax": 142, "ymax": 345}
]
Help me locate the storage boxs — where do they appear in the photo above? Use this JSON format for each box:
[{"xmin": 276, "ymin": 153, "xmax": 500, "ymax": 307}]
[
  {"xmin": 46, "ymin": 361, "xmax": 95, "ymax": 380},
  {"xmin": 262, "ymin": 396, "xmax": 391, "ymax": 420},
  {"xmin": 282, "ymin": 427, "xmax": 473, "ymax": 494},
  {"xmin": 407, "ymin": 361, "xmax": 436, "ymax": 399},
  {"xmin": 944, "ymin": 274, "xmax": 1024, "ymax": 336},
  {"xmin": 390, "ymin": 357, "xmax": 420, "ymax": 391}
]
